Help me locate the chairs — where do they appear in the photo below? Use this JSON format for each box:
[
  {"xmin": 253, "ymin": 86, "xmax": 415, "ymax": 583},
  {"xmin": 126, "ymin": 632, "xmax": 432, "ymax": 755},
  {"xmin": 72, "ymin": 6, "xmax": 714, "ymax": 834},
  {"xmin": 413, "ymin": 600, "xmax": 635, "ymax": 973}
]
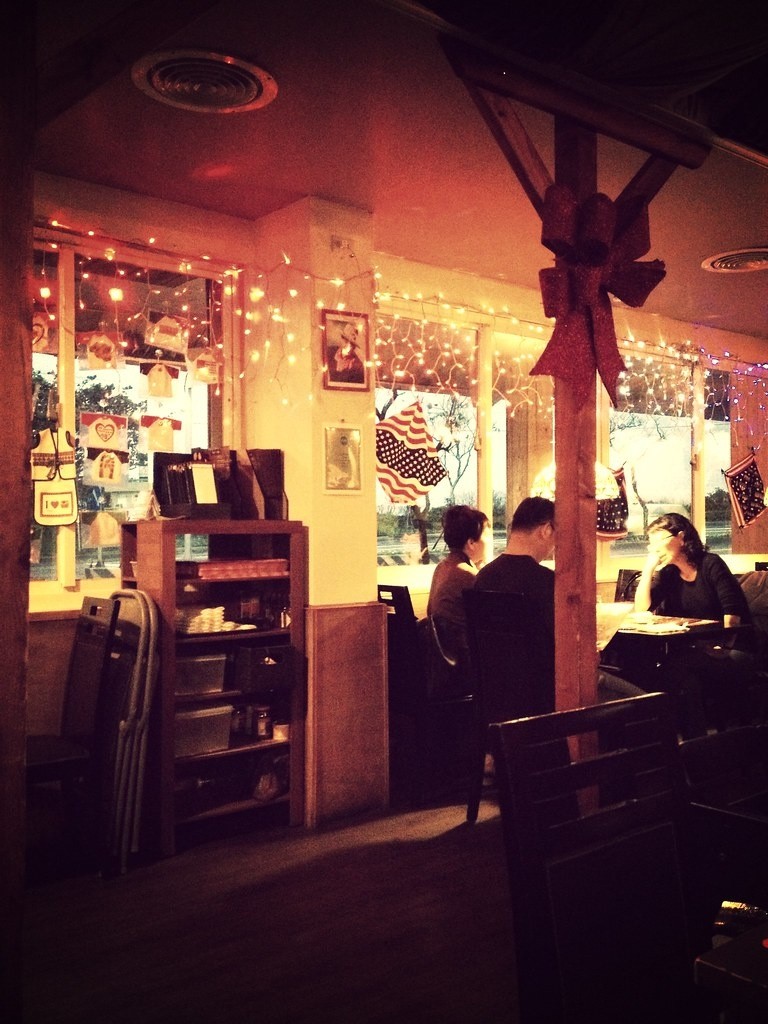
[
  {"xmin": 376, "ymin": 560, "xmax": 768, "ymax": 1024},
  {"xmin": 24, "ymin": 587, "xmax": 161, "ymax": 878}
]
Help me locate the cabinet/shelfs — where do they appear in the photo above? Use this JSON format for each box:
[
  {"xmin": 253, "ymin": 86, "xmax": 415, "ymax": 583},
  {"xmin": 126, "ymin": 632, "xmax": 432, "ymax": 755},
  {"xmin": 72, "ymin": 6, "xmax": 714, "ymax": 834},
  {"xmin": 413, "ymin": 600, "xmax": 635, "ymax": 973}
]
[{"xmin": 120, "ymin": 518, "xmax": 303, "ymax": 857}]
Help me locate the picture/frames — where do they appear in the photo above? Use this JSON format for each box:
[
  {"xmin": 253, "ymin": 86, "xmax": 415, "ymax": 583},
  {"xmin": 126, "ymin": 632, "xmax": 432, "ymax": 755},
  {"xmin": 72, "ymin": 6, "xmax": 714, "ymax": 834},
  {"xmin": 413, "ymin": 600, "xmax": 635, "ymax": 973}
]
[
  {"xmin": 322, "ymin": 423, "xmax": 365, "ymax": 496},
  {"xmin": 321, "ymin": 309, "xmax": 371, "ymax": 392}
]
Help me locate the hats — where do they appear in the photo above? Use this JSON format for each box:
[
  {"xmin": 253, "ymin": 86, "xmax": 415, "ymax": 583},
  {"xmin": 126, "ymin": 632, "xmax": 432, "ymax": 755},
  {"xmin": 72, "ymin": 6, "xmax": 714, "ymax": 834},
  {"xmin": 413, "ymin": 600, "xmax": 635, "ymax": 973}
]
[{"xmin": 341, "ymin": 325, "xmax": 362, "ymax": 347}]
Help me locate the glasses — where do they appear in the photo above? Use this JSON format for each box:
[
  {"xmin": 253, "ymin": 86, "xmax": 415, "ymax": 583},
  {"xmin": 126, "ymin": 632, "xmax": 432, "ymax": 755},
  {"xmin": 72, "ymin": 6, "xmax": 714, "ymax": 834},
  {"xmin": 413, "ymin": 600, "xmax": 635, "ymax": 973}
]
[{"xmin": 647, "ymin": 534, "xmax": 675, "ymax": 551}]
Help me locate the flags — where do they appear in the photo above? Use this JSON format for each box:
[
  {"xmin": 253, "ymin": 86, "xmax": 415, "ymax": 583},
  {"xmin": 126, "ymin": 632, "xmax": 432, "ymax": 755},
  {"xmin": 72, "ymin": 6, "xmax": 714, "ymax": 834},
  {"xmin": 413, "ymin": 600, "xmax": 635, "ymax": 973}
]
[{"xmin": 375, "ymin": 400, "xmax": 449, "ymax": 503}]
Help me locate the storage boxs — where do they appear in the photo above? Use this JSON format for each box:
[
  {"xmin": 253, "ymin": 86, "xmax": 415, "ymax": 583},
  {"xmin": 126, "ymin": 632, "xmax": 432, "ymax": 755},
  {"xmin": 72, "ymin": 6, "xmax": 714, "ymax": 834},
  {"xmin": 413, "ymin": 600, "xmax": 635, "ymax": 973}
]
[
  {"xmin": 176, "ymin": 653, "xmax": 227, "ymax": 695},
  {"xmin": 174, "ymin": 703, "xmax": 236, "ymax": 757},
  {"xmin": 234, "ymin": 640, "xmax": 296, "ymax": 691}
]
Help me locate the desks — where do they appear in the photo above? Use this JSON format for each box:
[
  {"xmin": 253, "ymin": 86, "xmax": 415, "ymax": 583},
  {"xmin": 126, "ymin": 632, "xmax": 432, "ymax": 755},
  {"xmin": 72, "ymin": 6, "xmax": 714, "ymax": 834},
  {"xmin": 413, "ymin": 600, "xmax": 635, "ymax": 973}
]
[{"xmin": 608, "ymin": 613, "xmax": 724, "ymax": 739}]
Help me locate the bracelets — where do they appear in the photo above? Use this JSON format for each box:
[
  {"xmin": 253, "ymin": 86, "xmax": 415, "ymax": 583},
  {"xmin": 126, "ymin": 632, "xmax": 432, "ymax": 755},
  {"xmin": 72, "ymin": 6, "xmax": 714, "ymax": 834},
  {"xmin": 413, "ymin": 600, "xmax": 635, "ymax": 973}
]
[{"xmin": 722, "ymin": 644, "xmax": 734, "ymax": 650}]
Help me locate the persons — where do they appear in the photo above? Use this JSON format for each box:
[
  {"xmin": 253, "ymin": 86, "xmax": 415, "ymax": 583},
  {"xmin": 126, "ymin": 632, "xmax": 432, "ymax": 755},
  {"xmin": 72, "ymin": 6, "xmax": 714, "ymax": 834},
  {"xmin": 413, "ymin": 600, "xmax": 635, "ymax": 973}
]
[
  {"xmin": 326, "ymin": 323, "xmax": 364, "ymax": 384},
  {"xmin": 635, "ymin": 512, "xmax": 761, "ymax": 659},
  {"xmin": 426, "ymin": 495, "xmax": 646, "ymax": 713}
]
